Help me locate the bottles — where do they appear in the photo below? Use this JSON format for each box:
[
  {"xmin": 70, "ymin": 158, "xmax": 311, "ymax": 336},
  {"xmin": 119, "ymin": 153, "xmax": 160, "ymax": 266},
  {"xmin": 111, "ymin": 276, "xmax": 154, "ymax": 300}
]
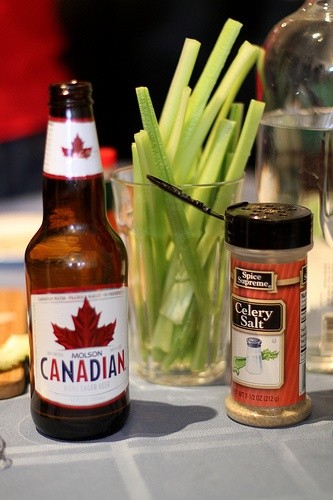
[
  {"xmin": 24, "ymin": 81, "xmax": 131, "ymax": 442},
  {"xmin": 257, "ymin": 0, "xmax": 333, "ymax": 373},
  {"xmin": 223, "ymin": 200, "xmax": 314, "ymax": 428}
]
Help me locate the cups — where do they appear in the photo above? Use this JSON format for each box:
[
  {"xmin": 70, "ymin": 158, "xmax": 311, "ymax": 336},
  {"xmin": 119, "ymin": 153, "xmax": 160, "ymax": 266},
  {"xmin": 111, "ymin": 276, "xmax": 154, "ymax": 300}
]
[{"xmin": 104, "ymin": 166, "xmax": 247, "ymax": 385}]
